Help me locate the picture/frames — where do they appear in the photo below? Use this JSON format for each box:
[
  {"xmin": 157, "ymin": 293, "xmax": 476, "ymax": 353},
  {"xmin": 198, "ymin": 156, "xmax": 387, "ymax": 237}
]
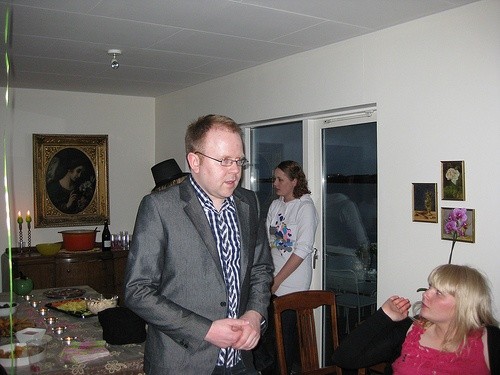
[
  {"xmin": 31, "ymin": 133, "xmax": 111, "ymax": 230},
  {"xmin": 440, "ymin": 160, "xmax": 466, "ymax": 201},
  {"xmin": 411, "ymin": 182, "xmax": 438, "ymax": 223},
  {"xmin": 440, "ymin": 207, "xmax": 475, "ymax": 243}
]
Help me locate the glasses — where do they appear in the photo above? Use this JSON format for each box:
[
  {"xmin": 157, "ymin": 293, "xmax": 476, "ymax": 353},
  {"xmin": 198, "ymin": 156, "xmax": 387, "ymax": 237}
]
[{"xmin": 193, "ymin": 152, "xmax": 249, "ymax": 166}]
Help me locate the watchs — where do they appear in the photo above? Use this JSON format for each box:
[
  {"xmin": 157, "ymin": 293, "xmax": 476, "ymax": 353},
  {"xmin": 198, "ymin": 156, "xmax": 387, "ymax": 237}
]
[{"xmin": 259, "ymin": 317, "xmax": 266, "ymax": 327}]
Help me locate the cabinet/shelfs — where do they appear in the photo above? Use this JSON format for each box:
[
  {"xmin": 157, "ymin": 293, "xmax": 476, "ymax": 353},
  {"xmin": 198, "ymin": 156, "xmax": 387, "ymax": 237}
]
[
  {"xmin": 111, "ymin": 246, "xmax": 129, "ymax": 300},
  {"xmin": 56, "ymin": 243, "xmax": 115, "ymax": 298},
  {"xmin": 12, "ymin": 251, "xmax": 56, "ymax": 290}
]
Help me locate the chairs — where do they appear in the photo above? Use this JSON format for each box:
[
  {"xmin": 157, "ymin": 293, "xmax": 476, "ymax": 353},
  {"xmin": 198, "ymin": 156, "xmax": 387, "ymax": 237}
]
[
  {"xmin": 326, "ymin": 267, "xmax": 377, "ymax": 336},
  {"xmin": 271, "ymin": 291, "xmax": 341, "ymax": 375}
]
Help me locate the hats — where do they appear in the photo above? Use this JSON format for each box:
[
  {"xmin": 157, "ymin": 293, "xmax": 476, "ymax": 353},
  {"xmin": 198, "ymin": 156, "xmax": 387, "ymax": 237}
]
[{"xmin": 151, "ymin": 159, "xmax": 191, "ymax": 191}]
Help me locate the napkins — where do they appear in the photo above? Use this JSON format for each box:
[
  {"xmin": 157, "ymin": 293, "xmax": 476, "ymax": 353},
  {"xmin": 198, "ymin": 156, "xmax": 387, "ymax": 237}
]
[{"xmin": 67, "ymin": 347, "xmax": 109, "ymax": 365}]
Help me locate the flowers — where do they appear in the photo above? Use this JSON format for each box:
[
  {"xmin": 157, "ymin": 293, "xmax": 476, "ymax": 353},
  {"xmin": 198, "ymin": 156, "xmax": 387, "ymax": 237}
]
[{"xmin": 416, "ymin": 208, "xmax": 468, "ymax": 293}]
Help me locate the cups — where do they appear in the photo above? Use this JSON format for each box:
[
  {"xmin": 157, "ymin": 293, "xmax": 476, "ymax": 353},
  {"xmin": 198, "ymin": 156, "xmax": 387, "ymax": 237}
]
[
  {"xmin": 111, "ymin": 231, "xmax": 130, "ymax": 250},
  {"xmin": 26, "ymin": 338, "xmax": 47, "ymax": 375},
  {"xmin": 13, "ymin": 277, "xmax": 33, "ymax": 296}
]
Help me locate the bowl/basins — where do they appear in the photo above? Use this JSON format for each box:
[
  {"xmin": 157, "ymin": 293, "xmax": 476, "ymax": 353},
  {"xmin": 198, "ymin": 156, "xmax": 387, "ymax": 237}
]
[
  {"xmin": 0, "ymin": 301, "xmax": 19, "ymax": 316},
  {"xmin": 15, "ymin": 328, "xmax": 46, "ymax": 343},
  {"xmin": 36, "ymin": 243, "xmax": 62, "ymax": 256}
]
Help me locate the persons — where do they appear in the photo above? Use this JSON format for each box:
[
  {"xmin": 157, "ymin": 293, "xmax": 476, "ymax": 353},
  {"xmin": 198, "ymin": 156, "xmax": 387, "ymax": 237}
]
[
  {"xmin": 332, "ymin": 263, "xmax": 500, "ymax": 375},
  {"xmin": 149, "ymin": 159, "xmax": 191, "ymax": 195},
  {"xmin": 120, "ymin": 114, "xmax": 275, "ymax": 375},
  {"xmin": 254, "ymin": 160, "xmax": 318, "ymax": 375}
]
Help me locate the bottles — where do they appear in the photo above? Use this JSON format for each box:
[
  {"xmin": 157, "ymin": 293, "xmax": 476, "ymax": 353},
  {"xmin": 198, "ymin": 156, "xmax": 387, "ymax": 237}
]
[{"xmin": 102, "ymin": 219, "xmax": 112, "ymax": 251}]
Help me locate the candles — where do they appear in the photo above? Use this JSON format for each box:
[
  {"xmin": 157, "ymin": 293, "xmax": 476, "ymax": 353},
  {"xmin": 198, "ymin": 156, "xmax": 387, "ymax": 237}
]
[
  {"xmin": 26, "ymin": 209, "xmax": 32, "ymax": 223},
  {"xmin": 16, "ymin": 210, "xmax": 24, "ymax": 223}
]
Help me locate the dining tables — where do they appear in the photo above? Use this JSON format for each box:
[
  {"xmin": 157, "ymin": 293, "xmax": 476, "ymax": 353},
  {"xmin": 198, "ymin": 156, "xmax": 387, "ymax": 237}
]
[{"xmin": 0, "ymin": 284, "xmax": 148, "ymax": 375}]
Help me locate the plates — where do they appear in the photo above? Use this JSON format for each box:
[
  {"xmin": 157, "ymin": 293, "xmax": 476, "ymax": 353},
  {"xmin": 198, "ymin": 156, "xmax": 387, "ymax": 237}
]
[{"xmin": 0, "ymin": 342, "xmax": 45, "ymax": 367}]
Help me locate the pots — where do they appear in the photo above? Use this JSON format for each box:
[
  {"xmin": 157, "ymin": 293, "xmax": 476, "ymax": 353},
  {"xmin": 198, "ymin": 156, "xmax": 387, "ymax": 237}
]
[{"xmin": 58, "ymin": 229, "xmax": 100, "ymax": 251}]
